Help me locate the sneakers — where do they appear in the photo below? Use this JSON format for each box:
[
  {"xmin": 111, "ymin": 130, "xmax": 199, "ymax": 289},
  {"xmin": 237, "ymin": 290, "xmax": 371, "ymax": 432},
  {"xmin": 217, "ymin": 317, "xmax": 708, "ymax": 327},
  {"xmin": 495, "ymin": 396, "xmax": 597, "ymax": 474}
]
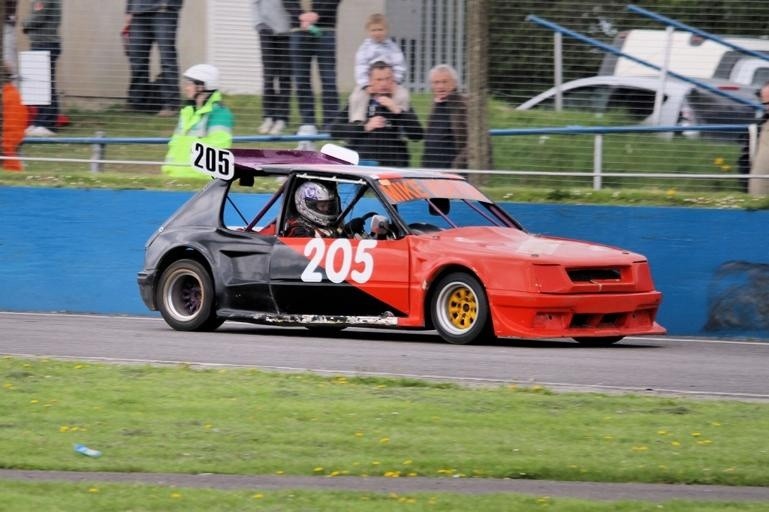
[
  {"xmin": 23, "ymin": 125, "xmax": 56, "ymax": 137},
  {"xmin": 257, "ymin": 117, "xmax": 288, "ymax": 134}
]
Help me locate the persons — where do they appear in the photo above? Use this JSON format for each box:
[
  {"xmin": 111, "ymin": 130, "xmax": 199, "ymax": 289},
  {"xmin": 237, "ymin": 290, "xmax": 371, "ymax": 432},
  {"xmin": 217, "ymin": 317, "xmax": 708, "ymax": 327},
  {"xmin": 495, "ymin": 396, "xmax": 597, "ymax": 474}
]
[
  {"xmin": 0, "ymin": 0, "xmax": 19, "ymax": 91},
  {"xmin": 160, "ymin": 63, "xmax": 236, "ymax": 181},
  {"xmin": 745, "ymin": 82, "xmax": 769, "ymax": 197},
  {"xmin": 123, "ymin": 1, "xmax": 184, "ymax": 118},
  {"xmin": 251, "ymin": 0, "xmax": 470, "ymax": 171},
  {"xmin": 24, "ymin": 0, "xmax": 63, "ymax": 137},
  {"xmin": 284, "ymin": 181, "xmax": 367, "ymax": 239}
]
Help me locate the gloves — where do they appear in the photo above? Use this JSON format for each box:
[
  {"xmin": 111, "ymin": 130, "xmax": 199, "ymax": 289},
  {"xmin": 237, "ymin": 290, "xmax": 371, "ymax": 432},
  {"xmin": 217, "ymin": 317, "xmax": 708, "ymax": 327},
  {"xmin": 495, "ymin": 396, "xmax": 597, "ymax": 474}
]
[{"xmin": 345, "ymin": 218, "xmax": 365, "ymax": 234}]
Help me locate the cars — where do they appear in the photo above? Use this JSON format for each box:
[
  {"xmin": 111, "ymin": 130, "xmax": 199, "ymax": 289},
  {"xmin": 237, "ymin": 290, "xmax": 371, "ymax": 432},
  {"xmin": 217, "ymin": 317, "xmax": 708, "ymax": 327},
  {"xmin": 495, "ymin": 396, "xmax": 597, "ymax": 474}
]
[
  {"xmin": 138, "ymin": 140, "xmax": 667, "ymax": 345},
  {"xmin": 516, "ymin": 75, "xmax": 763, "ymax": 139}
]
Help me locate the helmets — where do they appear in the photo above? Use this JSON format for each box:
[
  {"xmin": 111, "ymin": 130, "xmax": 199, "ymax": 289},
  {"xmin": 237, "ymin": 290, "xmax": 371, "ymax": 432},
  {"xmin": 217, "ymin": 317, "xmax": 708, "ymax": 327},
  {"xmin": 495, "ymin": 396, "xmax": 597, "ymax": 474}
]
[
  {"xmin": 182, "ymin": 63, "xmax": 218, "ymax": 91},
  {"xmin": 294, "ymin": 182, "xmax": 337, "ymax": 226}
]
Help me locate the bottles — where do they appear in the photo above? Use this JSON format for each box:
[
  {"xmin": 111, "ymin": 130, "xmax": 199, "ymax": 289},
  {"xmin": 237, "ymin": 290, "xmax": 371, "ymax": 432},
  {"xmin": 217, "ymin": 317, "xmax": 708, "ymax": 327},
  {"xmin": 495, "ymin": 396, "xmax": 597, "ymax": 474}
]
[{"xmin": 307, "ymin": 24, "xmax": 322, "ymax": 38}]
